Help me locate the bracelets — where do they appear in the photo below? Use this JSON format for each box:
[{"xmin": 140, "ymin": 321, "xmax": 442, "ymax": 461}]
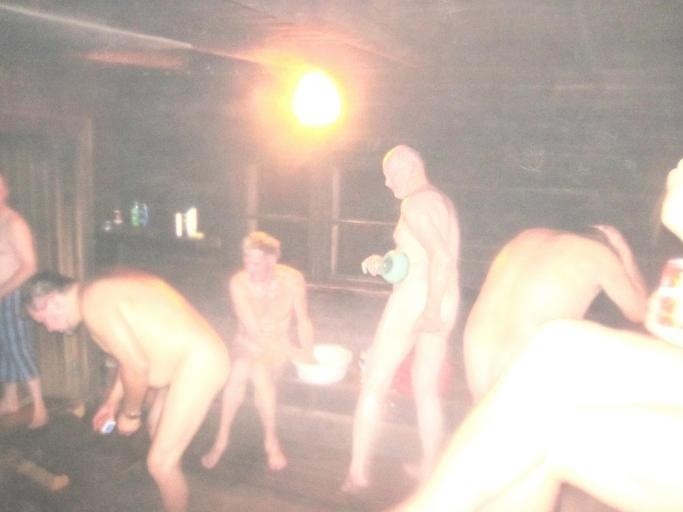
[{"xmin": 121, "ymin": 409, "xmax": 141, "ymax": 420}]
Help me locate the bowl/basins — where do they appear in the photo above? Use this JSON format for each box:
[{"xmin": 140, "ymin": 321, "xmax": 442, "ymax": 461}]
[{"xmin": 293, "ymin": 342, "xmax": 353, "ymax": 385}]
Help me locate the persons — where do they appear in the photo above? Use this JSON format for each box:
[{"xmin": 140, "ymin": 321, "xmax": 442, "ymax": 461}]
[
  {"xmin": 460, "ymin": 223, "xmax": 650, "ymax": 411},
  {"xmin": 21, "ymin": 270, "xmax": 230, "ymax": 511},
  {"xmin": 0, "ymin": 171, "xmax": 48, "ymax": 430},
  {"xmin": 336, "ymin": 144, "xmax": 461, "ymax": 493},
  {"xmin": 377, "ymin": 158, "xmax": 681, "ymax": 510},
  {"xmin": 199, "ymin": 230, "xmax": 315, "ymax": 471}
]
